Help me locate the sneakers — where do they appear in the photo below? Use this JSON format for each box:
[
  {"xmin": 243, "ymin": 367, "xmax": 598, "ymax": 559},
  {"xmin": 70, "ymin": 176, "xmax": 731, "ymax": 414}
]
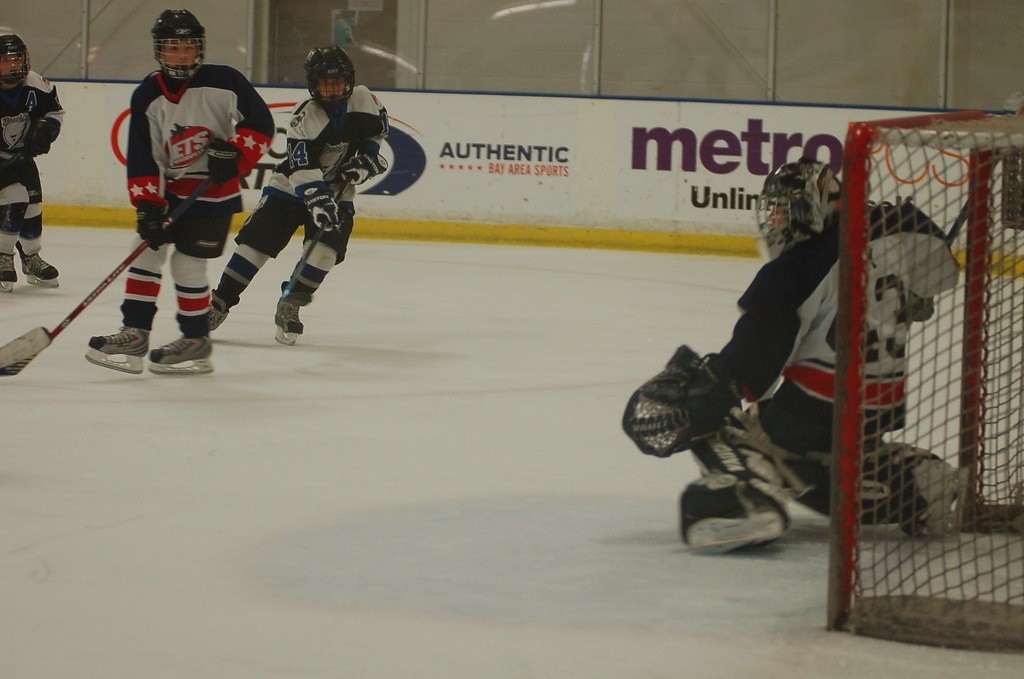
[
  {"xmin": 0, "ymin": 251, "xmax": 17, "ymax": 292},
  {"xmin": 15, "ymin": 240, "xmax": 59, "ymax": 289},
  {"xmin": 149, "ymin": 334, "xmax": 214, "ymax": 375},
  {"xmin": 275, "ymin": 301, "xmax": 304, "ymax": 346},
  {"xmin": 85, "ymin": 325, "xmax": 150, "ymax": 374},
  {"xmin": 208, "ymin": 289, "xmax": 229, "ymax": 331}
]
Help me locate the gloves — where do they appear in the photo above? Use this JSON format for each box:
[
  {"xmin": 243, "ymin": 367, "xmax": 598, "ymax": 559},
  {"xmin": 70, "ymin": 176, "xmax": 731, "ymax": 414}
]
[
  {"xmin": 136, "ymin": 199, "xmax": 177, "ymax": 250},
  {"xmin": 23, "ymin": 117, "xmax": 61, "ymax": 157},
  {"xmin": 339, "ymin": 152, "xmax": 388, "ymax": 186},
  {"xmin": 303, "ymin": 186, "xmax": 344, "ymax": 232},
  {"xmin": 207, "ymin": 138, "xmax": 243, "ymax": 187}
]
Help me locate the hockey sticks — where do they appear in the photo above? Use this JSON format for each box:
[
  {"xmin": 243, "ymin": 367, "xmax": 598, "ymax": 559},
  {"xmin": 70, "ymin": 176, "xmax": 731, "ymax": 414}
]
[
  {"xmin": 281, "ymin": 171, "xmax": 356, "ymax": 305},
  {"xmin": 944, "ymin": 195, "xmax": 971, "ymax": 246},
  {"xmin": 0, "ymin": 175, "xmax": 214, "ymax": 377}
]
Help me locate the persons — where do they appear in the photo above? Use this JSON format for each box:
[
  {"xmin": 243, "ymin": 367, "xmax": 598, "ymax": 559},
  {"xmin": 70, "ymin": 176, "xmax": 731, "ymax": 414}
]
[
  {"xmin": 679, "ymin": 162, "xmax": 961, "ymax": 555},
  {"xmin": 205, "ymin": 44, "xmax": 390, "ymax": 346},
  {"xmin": 0, "ymin": 33, "xmax": 66, "ymax": 292},
  {"xmin": 85, "ymin": 10, "xmax": 276, "ymax": 376}
]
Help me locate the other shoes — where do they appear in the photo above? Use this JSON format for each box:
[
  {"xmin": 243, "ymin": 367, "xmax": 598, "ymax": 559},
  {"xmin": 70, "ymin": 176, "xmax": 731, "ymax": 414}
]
[{"xmin": 686, "ymin": 514, "xmax": 788, "ymax": 555}]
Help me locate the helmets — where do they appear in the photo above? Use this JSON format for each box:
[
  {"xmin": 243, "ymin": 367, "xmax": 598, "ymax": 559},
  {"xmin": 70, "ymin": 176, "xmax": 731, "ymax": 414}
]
[
  {"xmin": 303, "ymin": 45, "xmax": 356, "ymax": 106},
  {"xmin": 0, "ymin": 34, "xmax": 31, "ymax": 83},
  {"xmin": 150, "ymin": 9, "xmax": 209, "ymax": 80},
  {"xmin": 755, "ymin": 159, "xmax": 842, "ymax": 262}
]
